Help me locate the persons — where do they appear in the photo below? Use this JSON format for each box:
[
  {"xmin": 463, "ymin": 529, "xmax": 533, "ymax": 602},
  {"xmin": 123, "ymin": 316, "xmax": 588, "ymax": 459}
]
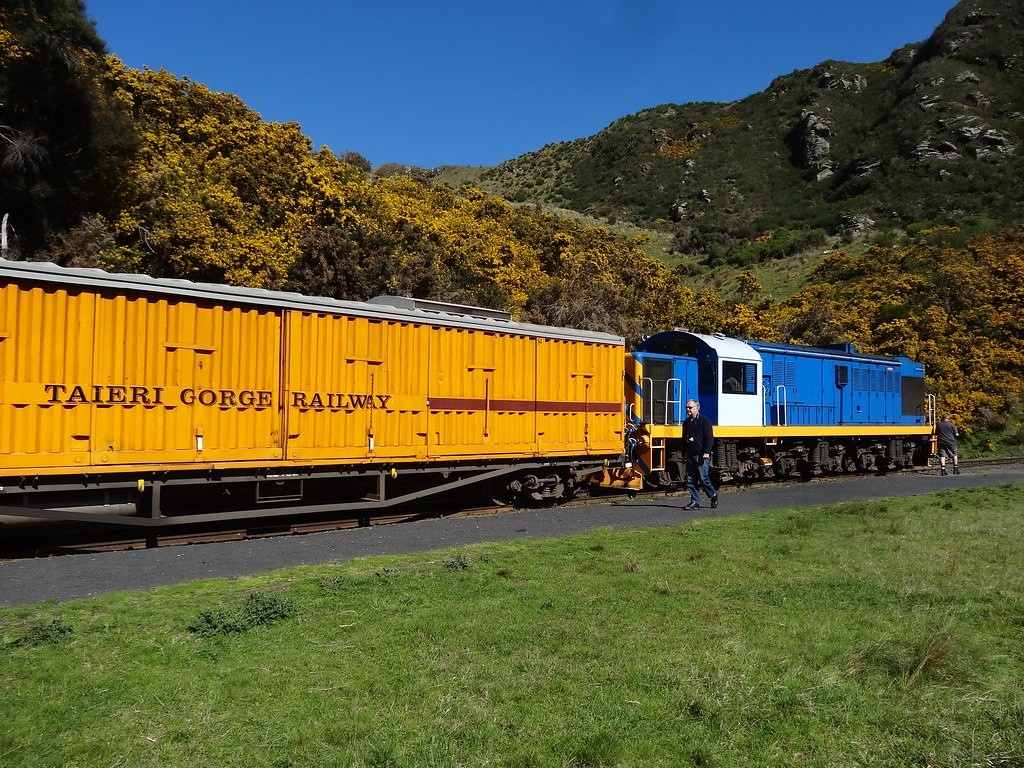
[
  {"xmin": 683, "ymin": 400, "xmax": 718, "ymax": 509},
  {"xmin": 935, "ymin": 413, "xmax": 961, "ymax": 475}
]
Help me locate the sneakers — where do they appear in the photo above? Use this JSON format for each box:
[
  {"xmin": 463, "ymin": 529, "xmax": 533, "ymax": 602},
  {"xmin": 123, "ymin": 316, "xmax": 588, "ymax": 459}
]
[
  {"xmin": 941, "ymin": 469, "xmax": 948, "ymax": 476},
  {"xmin": 711, "ymin": 492, "xmax": 718, "ymax": 508},
  {"xmin": 953, "ymin": 467, "xmax": 961, "ymax": 475},
  {"xmin": 684, "ymin": 501, "xmax": 700, "ymax": 511}
]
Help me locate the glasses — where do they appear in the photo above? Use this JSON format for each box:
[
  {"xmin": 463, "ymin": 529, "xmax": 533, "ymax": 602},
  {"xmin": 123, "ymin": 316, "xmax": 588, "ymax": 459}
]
[{"xmin": 685, "ymin": 405, "xmax": 700, "ymax": 409}]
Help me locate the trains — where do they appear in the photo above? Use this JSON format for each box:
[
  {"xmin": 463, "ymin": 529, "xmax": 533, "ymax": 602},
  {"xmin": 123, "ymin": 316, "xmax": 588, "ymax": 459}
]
[{"xmin": 1, "ymin": 252, "xmax": 939, "ymax": 573}]
